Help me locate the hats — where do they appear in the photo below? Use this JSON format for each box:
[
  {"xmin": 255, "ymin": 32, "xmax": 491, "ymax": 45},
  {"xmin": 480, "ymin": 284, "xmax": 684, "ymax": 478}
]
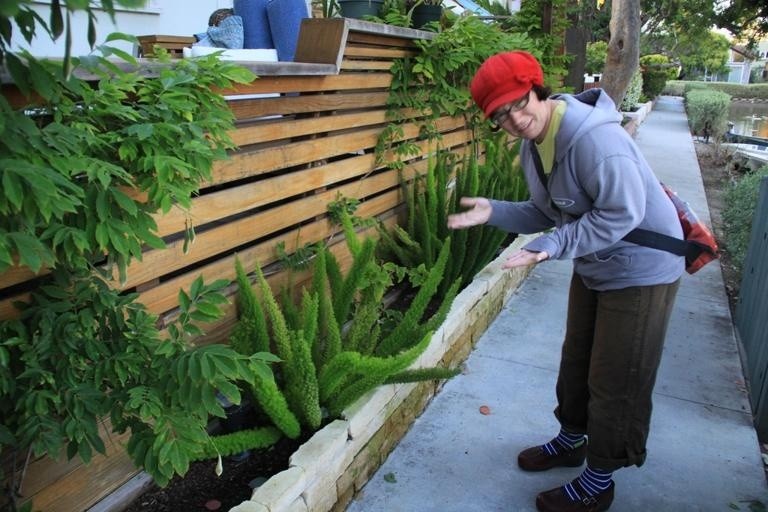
[{"xmin": 470, "ymin": 50, "xmax": 544, "ymax": 124}]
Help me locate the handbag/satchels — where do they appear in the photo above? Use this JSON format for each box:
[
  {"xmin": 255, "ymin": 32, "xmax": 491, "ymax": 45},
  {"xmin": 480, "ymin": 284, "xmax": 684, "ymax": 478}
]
[{"xmin": 660, "ymin": 181, "xmax": 721, "ymax": 275}]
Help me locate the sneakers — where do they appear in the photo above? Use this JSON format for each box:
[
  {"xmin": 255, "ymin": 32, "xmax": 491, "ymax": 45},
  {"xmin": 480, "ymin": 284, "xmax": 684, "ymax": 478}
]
[
  {"xmin": 537, "ymin": 475, "xmax": 615, "ymax": 512},
  {"xmin": 518, "ymin": 436, "xmax": 588, "ymax": 471}
]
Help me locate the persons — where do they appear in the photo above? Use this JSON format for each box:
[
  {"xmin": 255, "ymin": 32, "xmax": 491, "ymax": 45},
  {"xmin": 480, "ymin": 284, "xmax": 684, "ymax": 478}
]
[{"xmin": 443, "ymin": 50, "xmax": 689, "ymax": 511}]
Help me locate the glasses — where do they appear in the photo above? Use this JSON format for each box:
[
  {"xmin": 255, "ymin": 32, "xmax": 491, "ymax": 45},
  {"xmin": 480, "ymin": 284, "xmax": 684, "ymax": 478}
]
[{"xmin": 493, "ymin": 90, "xmax": 531, "ymax": 125}]
